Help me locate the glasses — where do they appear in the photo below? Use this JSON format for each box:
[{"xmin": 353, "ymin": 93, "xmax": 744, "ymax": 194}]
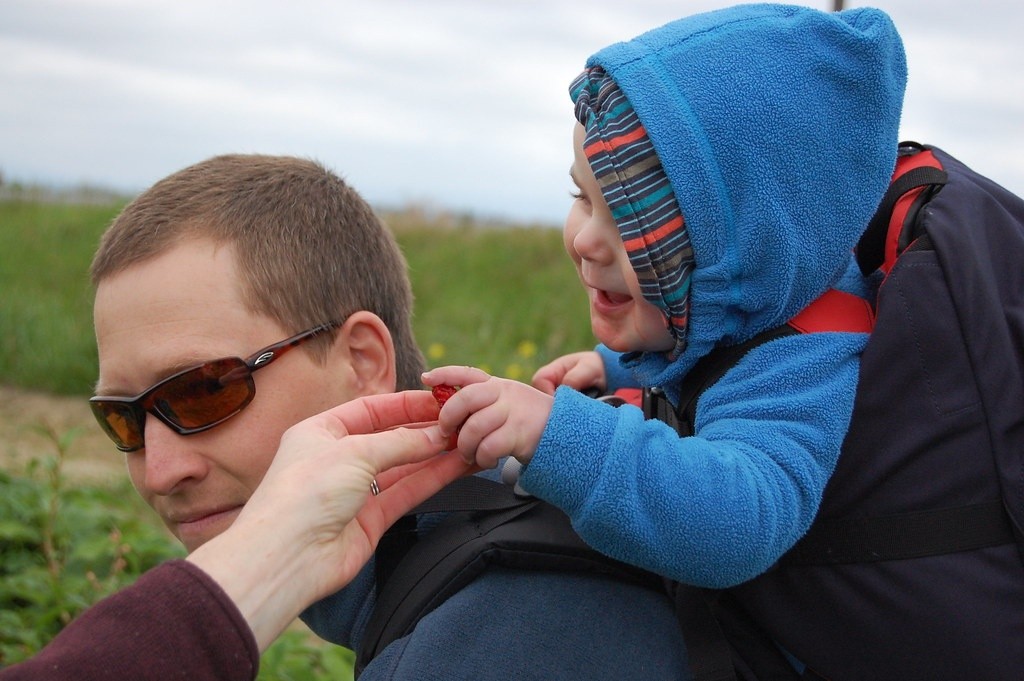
[{"xmin": 90, "ymin": 315, "xmax": 351, "ymax": 451}]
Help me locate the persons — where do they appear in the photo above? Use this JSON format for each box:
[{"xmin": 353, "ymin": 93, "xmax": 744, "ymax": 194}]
[
  {"xmin": 0, "ymin": 388, "xmax": 485, "ymax": 681},
  {"xmin": 420, "ymin": 1, "xmax": 1024, "ymax": 681},
  {"xmin": 84, "ymin": 152, "xmax": 799, "ymax": 681}
]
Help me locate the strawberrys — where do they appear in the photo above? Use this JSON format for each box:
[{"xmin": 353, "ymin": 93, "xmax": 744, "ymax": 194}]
[{"xmin": 431, "ymin": 385, "xmax": 473, "ymax": 433}]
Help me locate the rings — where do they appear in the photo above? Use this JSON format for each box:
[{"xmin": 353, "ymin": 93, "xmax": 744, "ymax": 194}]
[{"xmin": 370, "ymin": 479, "xmax": 379, "ymax": 495}]
